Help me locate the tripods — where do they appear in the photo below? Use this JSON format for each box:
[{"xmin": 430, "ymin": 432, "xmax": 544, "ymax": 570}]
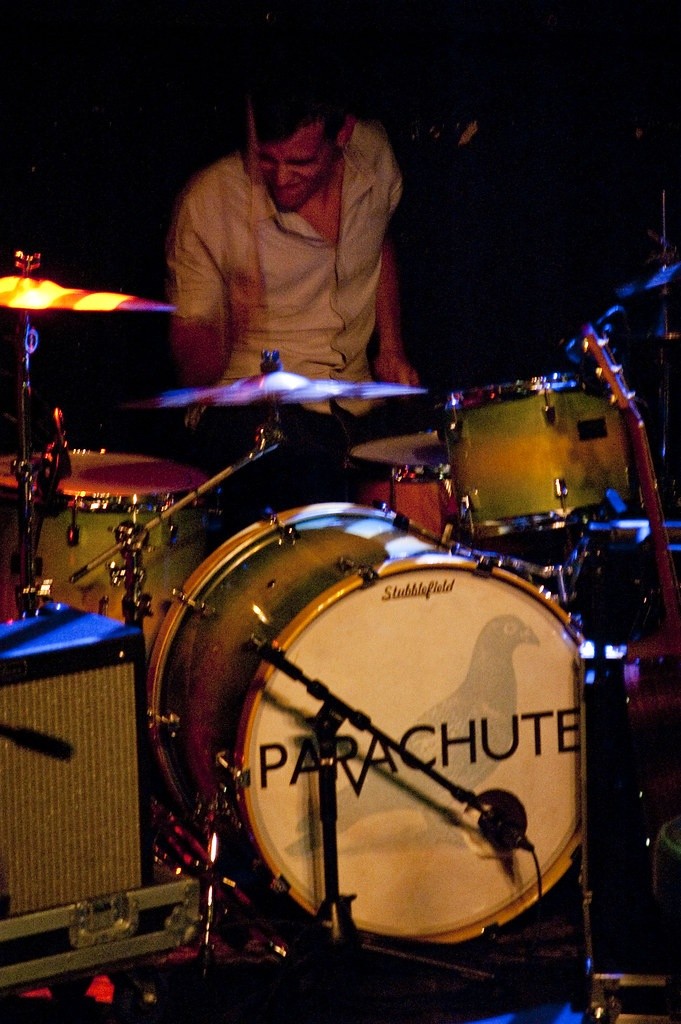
[{"xmin": 221, "ymin": 676, "xmax": 497, "ymax": 1019}]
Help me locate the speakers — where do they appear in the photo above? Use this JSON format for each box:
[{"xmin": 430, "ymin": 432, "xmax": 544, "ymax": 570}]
[{"xmin": 0, "ymin": 601, "xmax": 155, "ymax": 921}]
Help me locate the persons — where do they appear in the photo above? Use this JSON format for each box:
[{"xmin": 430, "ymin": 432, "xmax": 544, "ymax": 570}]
[{"xmin": 158, "ymin": 48, "xmax": 421, "ymax": 549}]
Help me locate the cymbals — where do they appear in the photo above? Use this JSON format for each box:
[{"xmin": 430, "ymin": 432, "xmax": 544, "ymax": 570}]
[
  {"xmin": 0, "ymin": 274, "xmax": 180, "ymax": 314},
  {"xmin": 117, "ymin": 364, "xmax": 423, "ymax": 415}
]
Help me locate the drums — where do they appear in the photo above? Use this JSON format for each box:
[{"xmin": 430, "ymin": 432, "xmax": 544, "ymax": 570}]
[
  {"xmin": 146, "ymin": 500, "xmax": 587, "ymax": 947},
  {"xmin": 451, "ymin": 379, "xmax": 636, "ymax": 538},
  {"xmin": 0, "ymin": 450, "xmax": 214, "ymax": 660},
  {"xmin": 343, "ymin": 428, "xmax": 460, "ymax": 542}
]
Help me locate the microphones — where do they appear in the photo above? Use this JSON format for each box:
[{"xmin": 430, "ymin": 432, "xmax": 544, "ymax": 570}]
[
  {"xmin": 476, "ymin": 811, "xmax": 534, "ymax": 853},
  {"xmin": 55, "ymin": 408, "xmax": 73, "ymax": 479},
  {"xmin": 566, "ymin": 306, "xmax": 627, "ymax": 363}
]
